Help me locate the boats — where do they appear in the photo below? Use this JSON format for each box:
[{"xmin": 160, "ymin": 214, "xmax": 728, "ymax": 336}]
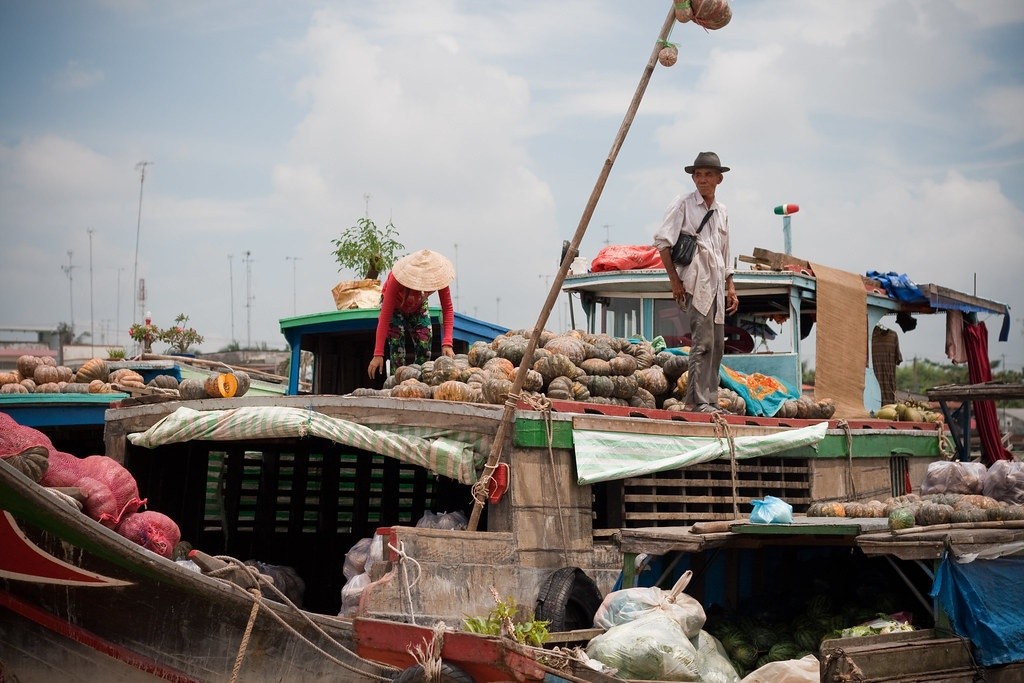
[
  {"xmin": 0, "ymin": 454, "xmax": 1024, "ymax": 682},
  {"xmin": 129, "ymin": 204, "xmax": 1023, "ymax": 617},
  {"xmin": 0, "ymin": 305, "xmax": 517, "ymax": 462}
]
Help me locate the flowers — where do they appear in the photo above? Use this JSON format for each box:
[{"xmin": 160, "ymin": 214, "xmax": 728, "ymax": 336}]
[
  {"xmin": 128, "ymin": 321, "xmax": 163, "ymax": 348},
  {"xmin": 163, "ymin": 313, "xmax": 204, "ymax": 353}
]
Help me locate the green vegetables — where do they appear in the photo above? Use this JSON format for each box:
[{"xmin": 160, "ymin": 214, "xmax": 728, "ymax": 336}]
[{"xmin": 459, "ymin": 597, "xmax": 553, "ymax": 649}]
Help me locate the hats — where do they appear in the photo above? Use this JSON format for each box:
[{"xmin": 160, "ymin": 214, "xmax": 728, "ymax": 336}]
[
  {"xmin": 683, "ymin": 152, "xmax": 731, "ymax": 175},
  {"xmin": 392, "ymin": 248, "xmax": 456, "ymax": 293}
]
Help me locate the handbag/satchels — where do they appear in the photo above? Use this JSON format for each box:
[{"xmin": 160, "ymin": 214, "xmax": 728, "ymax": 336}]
[
  {"xmin": 338, "ymin": 538, "xmax": 374, "ymax": 579},
  {"xmin": 919, "ymin": 455, "xmax": 986, "ymax": 496},
  {"xmin": 748, "ymin": 495, "xmax": 796, "ymax": 525},
  {"xmin": 689, "ymin": 631, "xmax": 740, "ymax": 683},
  {"xmin": 593, "ymin": 567, "xmax": 708, "ymax": 640},
  {"xmin": 579, "ymin": 617, "xmax": 697, "ymax": 683},
  {"xmin": 979, "ymin": 459, "xmax": 1024, "ymax": 505},
  {"xmin": 335, "ymin": 575, "xmax": 374, "ymax": 623},
  {"xmin": 661, "ymin": 201, "xmax": 720, "ymax": 269},
  {"xmin": 415, "ymin": 508, "xmax": 469, "ymax": 532}
]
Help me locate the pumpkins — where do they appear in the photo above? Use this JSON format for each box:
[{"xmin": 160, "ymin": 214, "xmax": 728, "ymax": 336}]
[
  {"xmin": 0, "ymin": 354, "xmax": 250, "ymax": 559},
  {"xmin": 807, "ymin": 493, "xmax": 1024, "ymax": 529},
  {"xmin": 353, "ymin": 329, "xmax": 836, "ymax": 419}
]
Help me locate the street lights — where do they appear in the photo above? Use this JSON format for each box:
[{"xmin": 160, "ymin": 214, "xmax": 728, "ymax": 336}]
[
  {"xmin": 57, "ymin": 162, "xmax": 301, "ymax": 353},
  {"xmin": 450, "ymin": 218, "xmax": 612, "ymax": 333}
]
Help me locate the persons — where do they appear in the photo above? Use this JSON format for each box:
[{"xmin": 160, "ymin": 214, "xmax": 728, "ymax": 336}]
[
  {"xmin": 653, "ymin": 152, "xmax": 740, "ymax": 415},
  {"xmin": 368, "ymin": 248, "xmax": 456, "ymax": 381}
]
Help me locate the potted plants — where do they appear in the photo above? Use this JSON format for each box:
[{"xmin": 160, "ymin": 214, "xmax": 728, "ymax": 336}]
[{"xmin": 329, "ymin": 218, "xmax": 407, "ymax": 314}]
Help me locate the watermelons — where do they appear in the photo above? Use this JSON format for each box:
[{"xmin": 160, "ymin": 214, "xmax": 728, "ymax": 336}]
[{"xmin": 706, "ymin": 594, "xmax": 872, "ymax": 677}]
